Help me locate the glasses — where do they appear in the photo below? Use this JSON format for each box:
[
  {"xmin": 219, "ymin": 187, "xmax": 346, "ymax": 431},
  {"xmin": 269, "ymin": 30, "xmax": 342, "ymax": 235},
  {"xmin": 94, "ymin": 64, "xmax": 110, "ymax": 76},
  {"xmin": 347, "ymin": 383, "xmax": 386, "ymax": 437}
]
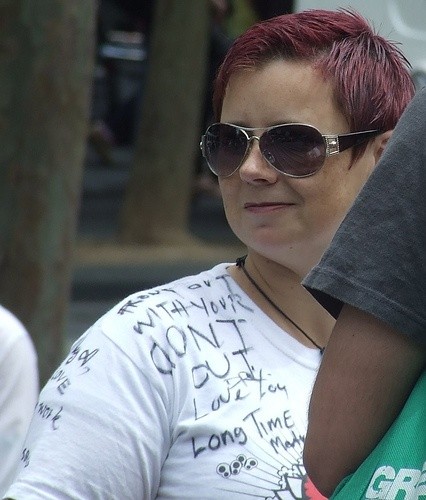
[{"xmin": 200, "ymin": 122, "xmax": 383, "ymax": 179}]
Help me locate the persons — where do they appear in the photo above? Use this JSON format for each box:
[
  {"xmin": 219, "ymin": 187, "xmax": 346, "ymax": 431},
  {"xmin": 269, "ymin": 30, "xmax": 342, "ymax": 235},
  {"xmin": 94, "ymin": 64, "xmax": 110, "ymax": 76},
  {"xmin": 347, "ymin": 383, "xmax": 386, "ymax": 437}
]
[
  {"xmin": 0, "ymin": 9, "xmax": 417, "ymax": 499},
  {"xmin": 299, "ymin": 81, "xmax": 426, "ymax": 500},
  {"xmin": 0, "ymin": 305, "xmax": 38, "ymax": 500}
]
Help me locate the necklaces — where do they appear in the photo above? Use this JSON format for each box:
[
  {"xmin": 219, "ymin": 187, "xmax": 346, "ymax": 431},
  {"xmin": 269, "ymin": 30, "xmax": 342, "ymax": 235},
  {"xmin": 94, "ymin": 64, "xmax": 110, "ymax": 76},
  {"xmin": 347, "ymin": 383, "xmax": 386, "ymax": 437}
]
[{"xmin": 234, "ymin": 253, "xmax": 326, "ymax": 356}]
